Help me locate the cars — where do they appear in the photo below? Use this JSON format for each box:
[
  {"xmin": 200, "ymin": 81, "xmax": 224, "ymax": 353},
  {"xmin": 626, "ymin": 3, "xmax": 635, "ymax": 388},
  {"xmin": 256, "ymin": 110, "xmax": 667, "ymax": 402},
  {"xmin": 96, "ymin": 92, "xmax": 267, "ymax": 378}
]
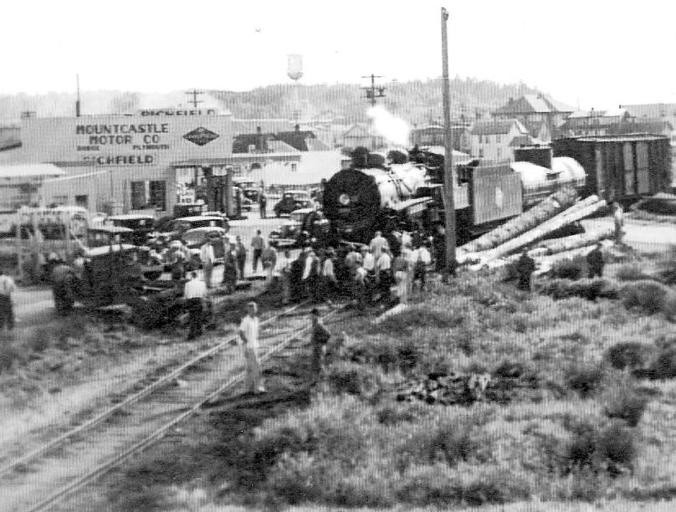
[
  {"xmin": 0, "ymin": 180, "xmax": 265, "ymax": 331},
  {"xmin": 271, "ymin": 190, "xmax": 314, "ymax": 217},
  {"xmin": 270, "ymin": 206, "xmax": 330, "ymax": 249}
]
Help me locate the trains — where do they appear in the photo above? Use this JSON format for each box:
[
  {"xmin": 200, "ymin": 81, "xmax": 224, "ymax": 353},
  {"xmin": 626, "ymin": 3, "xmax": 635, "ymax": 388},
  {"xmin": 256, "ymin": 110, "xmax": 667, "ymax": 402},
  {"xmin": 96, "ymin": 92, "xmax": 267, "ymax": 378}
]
[{"xmin": 309, "ymin": 134, "xmax": 676, "ymax": 257}]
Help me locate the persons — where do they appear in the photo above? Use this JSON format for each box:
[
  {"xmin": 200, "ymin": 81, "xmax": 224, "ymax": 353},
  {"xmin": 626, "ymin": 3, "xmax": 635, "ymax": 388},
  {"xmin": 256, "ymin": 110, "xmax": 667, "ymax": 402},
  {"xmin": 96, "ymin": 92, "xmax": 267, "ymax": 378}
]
[
  {"xmin": 586, "ymin": 244, "xmax": 606, "ymax": 279},
  {"xmin": 309, "ymin": 306, "xmax": 334, "ymax": 385},
  {"xmin": 518, "ymin": 247, "xmax": 536, "ymax": 291},
  {"xmin": 1, "ymin": 191, "xmax": 449, "ymax": 342},
  {"xmin": 237, "ymin": 298, "xmax": 270, "ymax": 396},
  {"xmin": 610, "ymin": 201, "xmax": 625, "ymax": 242}
]
